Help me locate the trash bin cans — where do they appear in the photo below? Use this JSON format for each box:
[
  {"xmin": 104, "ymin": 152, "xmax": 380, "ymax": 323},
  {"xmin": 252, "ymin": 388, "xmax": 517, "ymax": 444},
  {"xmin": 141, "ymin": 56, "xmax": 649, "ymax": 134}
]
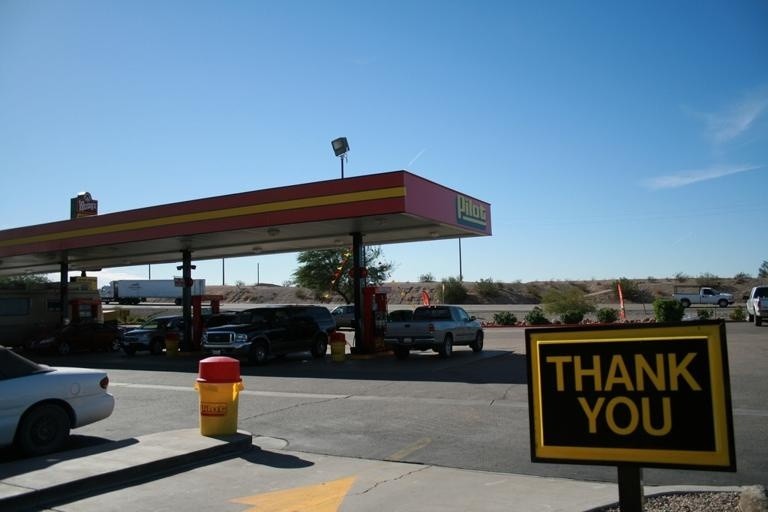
[
  {"xmin": 194, "ymin": 356, "xmax": 244, "ymax": 437},
  {"xmin": 329, "ymin": 333, "xmax": 347, "ymax": 362}
]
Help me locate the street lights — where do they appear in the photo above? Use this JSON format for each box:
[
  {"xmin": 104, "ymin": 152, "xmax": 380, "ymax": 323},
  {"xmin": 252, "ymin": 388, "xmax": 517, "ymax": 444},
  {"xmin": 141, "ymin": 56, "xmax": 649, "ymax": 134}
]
[{"xmin": 331, "ymin": 138, "xmax": 350, "ymax": 179}]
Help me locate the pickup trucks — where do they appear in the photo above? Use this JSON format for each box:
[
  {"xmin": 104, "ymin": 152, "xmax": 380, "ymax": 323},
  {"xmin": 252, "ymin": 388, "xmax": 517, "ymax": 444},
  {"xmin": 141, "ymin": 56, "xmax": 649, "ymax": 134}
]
[
  {"xmin": 671, "ymin": 287, "xmax": 735, "ymax": 307},
  {"xmin": 742, "ymin": 286, "xmax": 768, "ymax": 326},
  {"xmin": 384, "ymin": 305, "xmax": 484, "ymax": 357}
]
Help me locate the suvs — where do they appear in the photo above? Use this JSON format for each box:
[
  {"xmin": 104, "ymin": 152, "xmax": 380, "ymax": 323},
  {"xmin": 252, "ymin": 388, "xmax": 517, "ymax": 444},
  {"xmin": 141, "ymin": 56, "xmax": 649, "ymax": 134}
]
[{"xmin": 199, "ymin": 305, "xmax": 335, "ymax": 367}]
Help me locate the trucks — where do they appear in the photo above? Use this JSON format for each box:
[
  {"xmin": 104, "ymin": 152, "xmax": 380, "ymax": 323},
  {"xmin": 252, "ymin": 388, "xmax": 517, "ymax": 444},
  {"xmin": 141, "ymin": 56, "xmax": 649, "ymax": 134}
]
[{"xmin": 100, "ymin": 279, "xmax": 204, "ymax": 304}]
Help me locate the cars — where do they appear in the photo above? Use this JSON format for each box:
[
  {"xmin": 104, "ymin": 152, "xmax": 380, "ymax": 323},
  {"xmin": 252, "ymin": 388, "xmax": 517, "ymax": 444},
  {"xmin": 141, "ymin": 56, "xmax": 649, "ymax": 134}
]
[
  {"xmin": 0, "ymin": 345, "xmax": 115, "ymax": 464},
  {"xmin": 26, "ymin": 314, "xmax": 193, "ymax": 355},
  {"xmin": 331, "ymin": 305, "xmax": 355, "ymax": 329}
]
